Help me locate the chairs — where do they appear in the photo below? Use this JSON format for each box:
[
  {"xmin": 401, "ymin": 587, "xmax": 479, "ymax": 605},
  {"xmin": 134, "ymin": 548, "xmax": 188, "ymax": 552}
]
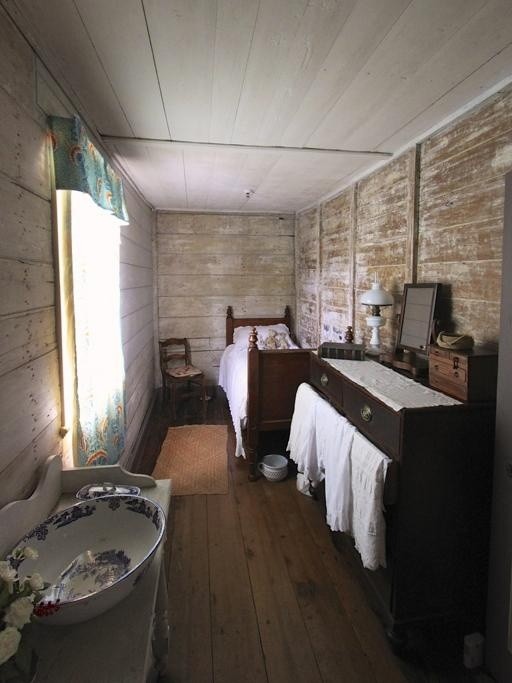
[{"xmin": 158, "ymin": 336, "xmax": 207, "ymax": 411}]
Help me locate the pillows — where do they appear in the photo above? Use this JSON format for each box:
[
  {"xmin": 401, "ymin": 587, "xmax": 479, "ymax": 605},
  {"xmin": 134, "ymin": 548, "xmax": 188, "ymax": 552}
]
[{"xmin": 232, "ymin": 323, "xmax": 297, "ymax": 350}]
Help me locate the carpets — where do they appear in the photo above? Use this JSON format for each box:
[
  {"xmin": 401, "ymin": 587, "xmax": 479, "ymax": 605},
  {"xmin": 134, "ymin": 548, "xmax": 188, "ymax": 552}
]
[{"xmin": 148, "ymin": 424, "xmax": 230, "ymax": 496}]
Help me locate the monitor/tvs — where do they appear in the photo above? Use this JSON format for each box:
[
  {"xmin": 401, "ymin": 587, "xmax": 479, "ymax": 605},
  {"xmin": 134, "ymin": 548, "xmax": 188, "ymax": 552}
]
[{"xmin": 395, "ymin": 283, "xmax": 441, "ymax": 355}]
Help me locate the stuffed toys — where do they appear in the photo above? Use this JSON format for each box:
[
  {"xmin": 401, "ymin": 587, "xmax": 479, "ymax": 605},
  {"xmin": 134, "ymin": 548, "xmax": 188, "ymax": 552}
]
[
  {"xmin": 279, "ymin": 331, "xmax": 289, "ymax": 349},
  {"xmin": 265, "ymin": 328, "xmax": 278, "ymax": 349}
]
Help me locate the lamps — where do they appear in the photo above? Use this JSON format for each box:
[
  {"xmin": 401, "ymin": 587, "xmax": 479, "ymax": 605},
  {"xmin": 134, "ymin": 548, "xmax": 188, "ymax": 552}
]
[{"xmin": 357, "ymin": 271, "xmax": 395, "ymax": 356}]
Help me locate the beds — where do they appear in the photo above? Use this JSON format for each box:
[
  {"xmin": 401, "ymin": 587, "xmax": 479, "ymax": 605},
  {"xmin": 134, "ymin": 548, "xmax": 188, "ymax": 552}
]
[{"xmin": 217, "ymin": 305, "xmax": 318, "ymax": 483}]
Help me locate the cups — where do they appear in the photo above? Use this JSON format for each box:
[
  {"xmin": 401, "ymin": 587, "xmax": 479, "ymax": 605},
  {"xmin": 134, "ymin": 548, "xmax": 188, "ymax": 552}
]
[{"xmin": 257, "ymin": 454, "xmax": 288, "ymax": 482}]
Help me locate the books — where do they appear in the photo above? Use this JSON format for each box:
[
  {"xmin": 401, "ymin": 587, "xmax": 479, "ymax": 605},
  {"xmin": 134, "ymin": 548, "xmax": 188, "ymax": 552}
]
[{"xmin": 316, "ymin": 341, "xmax": 368, "ymax": 361}]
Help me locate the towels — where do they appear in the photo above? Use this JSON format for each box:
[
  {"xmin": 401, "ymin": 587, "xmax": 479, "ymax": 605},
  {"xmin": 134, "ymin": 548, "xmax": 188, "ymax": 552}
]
[
  {"xmin": 285, "ymin": 381, "xmax": 391, "ymax": 572},
  {"xmin": 311, "ymin": 349, "xmax": 463, "ymax": 412}
]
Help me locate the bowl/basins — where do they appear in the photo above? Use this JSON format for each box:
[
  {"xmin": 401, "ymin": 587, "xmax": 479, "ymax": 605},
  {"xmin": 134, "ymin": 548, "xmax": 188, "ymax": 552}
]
[{"xmin": 3, "ymin": 494, "xmax": 167, "ymax": 628}]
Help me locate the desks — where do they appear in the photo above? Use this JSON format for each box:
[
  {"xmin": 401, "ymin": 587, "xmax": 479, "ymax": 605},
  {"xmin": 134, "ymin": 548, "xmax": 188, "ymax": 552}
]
[{"xmin": 0, "ymin": 454, "xmax": 174, "ymax": 682}]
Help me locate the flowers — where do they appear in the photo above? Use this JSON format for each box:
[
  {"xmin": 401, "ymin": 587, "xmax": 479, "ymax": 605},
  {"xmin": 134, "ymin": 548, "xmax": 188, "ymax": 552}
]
[{"xmin": 0, "ymin": 544, "xmax": 72, "ymax": 666}]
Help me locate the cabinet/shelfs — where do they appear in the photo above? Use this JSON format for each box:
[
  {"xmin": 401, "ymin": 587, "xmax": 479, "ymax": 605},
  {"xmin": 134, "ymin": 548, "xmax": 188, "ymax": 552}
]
[{"xmin": 302, "ymin": 338, "xmax": 494, "ymax": 681}]
